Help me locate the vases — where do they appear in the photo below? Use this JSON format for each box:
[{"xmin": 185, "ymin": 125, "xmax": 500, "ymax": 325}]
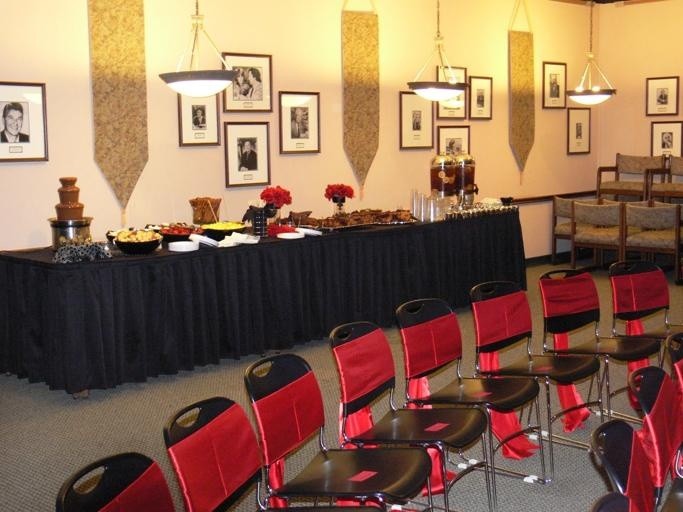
[{"xmin": 331, "ymin": 194, "xmax": 346, "ymax": 210}]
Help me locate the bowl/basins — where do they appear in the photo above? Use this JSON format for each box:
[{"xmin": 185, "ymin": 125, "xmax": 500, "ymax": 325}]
[{"xmin": 107, "ymin": 222, "xmax": 246, "ymax": 256}]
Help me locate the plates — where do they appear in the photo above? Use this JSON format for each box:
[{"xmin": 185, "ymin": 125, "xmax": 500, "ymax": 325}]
[{"xmin": 168, "ymin": 227, "xmax": 323, "ymax": 254}]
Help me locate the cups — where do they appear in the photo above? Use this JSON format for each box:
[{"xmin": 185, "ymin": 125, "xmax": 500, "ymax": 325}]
[
  {"xmin": 251, "ymin": 210, "xmax": 268, "ymax": 239},
  {"xmin": 408, "ymin": 188, "xmax": 435, "ymax": 223}
]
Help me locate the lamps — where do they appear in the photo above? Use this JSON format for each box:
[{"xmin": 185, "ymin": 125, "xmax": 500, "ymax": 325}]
[
  {"xmin": 406, "ymin": 0, "xmax": 471, "ymax": 103},
  {"xmin": 157, "ymin": 0, "xmax": 240, "ymax": 99},
  {"xmin": 564, "ymin": 2, "xmax": 617, "ymax": 107}
]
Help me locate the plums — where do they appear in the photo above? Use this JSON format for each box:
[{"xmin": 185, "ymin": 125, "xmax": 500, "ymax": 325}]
[{"xmin": 160, "ymin": 223, "xmax": 198, "ymax": 235}]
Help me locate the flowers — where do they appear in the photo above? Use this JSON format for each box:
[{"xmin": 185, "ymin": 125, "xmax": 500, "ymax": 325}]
[
  {"xmin": 259, "ymin": 186, "xmax": 292, "ymax": 208},
  {"xmin": 324, "ymin": 183, "xmax": 355, "ymax": 201}
]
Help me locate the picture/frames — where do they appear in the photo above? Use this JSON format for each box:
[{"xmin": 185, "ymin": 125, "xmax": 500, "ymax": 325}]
[
  {"xmin": 0, "ymin": 81, "xmax": 49, "ymax": 163},
  {"xmin": 221, "ymin": 52, "xmax": 273, "ymax": 113},
  {"xmin": 278, "ymin": 90, "xmax": 321, "ymax": 155},
  {"xmin": 398, "ymin": 64, "xmax": 494, "ymax": 158},
  {"xmin": 649, "ymin": 120, "xmax": 683, "ymax": 157},
  {"xmin": 644, "ymin": 75, "xmax": 680, "ymax": 117},
  {"xmin": 541, "ymin": 60, "xmax": 567, "ymax": 109},
  {"xmin": 176, "ymin": 92, "xmax": 221, "ymax": 147},
  {"xmin": 223, "ymin": 121, "xmax": 271, "ymax": 188},
  {"xmin": 566, "ymin": 108, "xmax": 592, "ymax": 156}
]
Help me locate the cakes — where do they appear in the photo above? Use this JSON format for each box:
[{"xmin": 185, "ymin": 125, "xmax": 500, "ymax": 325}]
[{"xmin": 55, "ymin": 177, "xmax": 83, "ymax": 220}]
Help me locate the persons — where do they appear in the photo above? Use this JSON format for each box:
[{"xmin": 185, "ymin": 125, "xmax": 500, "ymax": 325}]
[
  {"xmin": 576, "ymin": 124, "xmax": 582, "ymax": 139},
  {"xmin": 657, "ymin": 89, "xmax": 668, "ymax": 105},
  {"xmin": 412, "ymin": 112, "xmax": 422, "ymax": 131},
  {"xmin": 291, "ymin": 108, "xmax": 309, "ymax": 139},
  {"xmin": 550, "ymin": 76, "xmax": 560, "ymax": 98},
  {"xmin": 477, "ymin": 91, "xmax": 484, "ymax": 107},
  {"xmin": 449, "ymin": 139, "xmax": 458, "ymax": 155},
  {"xmin": 239, "ymin": 139, "xmax": 257, "ymax": 172},
  {"xmin": 0, "ymin": 103, "xmax": 29, "ymax": 142},
  {"xmin": 232, "ymin": 67, "xmax": 263, "ymax": 102},
  {"xmin": 662, "ymin": 133, "xmax": 673, "ymax": 149},
  {"xmin": 192, "ymin": 108, "xmax": 207, "ymax": 130}
]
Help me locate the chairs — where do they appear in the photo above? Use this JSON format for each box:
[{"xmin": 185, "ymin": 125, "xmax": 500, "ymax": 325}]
[
  {"xmin": 598, "ymin": 261, "xmax": 683, "ymax": 419},
  {"xmin": 459, "ymin": 279, "xmax": 604, "ymax": 482},
  {"xmin": 528, "ymin": 268, "xmax": 664, "ymax": 451},
  {"xmin": 395, "ymin": 298, "xmax": 547, "ymax": 512},
  {"xmin": 549, "ymin": 154, "xmax": 682, "ymax": 285},
  {"xmin": 162, "ymin": 396, "xmax": 386, "ymax": 512},
  {"xmin": 587, "ymin": 330, "xmax": 683, "ymax": 512},
  {"xmin": 55, "ymin": 452, "xmax": 173, "ymax": 512},
  {"xmin": 243, "ymin": 353, "xmax": 433, "ymax": 512},
  {"xmin": 329, "ymin": 322, "xmax": 494, "ymax": 512}
]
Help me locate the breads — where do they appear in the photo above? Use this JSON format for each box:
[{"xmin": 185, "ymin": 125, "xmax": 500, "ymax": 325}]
[{"xmin": 306, "ymin": 209, "xmax": 412, "ymax": 227}]
